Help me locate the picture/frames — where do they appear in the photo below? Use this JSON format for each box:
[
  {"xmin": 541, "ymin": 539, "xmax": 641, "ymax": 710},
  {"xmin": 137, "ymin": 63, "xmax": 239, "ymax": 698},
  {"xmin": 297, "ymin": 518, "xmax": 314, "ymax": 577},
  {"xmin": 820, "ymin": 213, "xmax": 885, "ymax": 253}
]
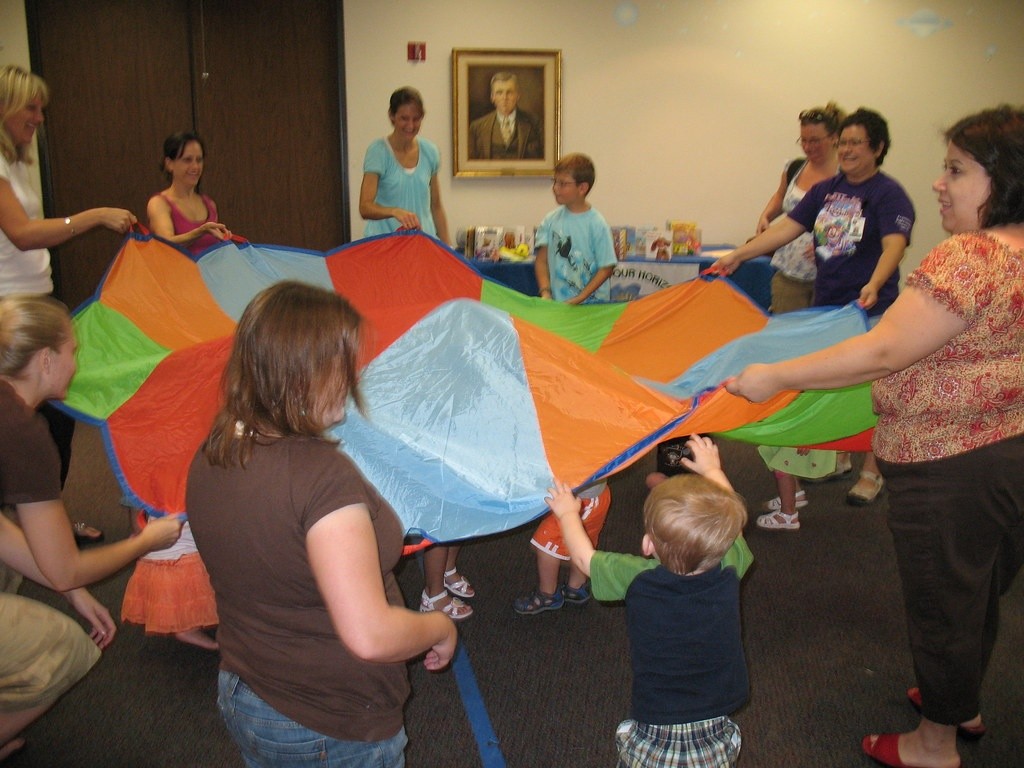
[{"xmin": 452, "ymin": 47, "xmax": 564, "ymax": 179}]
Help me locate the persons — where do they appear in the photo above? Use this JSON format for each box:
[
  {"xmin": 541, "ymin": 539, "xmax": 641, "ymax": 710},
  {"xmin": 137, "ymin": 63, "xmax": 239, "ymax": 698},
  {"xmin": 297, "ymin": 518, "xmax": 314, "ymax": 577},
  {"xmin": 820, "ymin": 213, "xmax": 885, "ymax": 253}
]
[
  {"xmin": 468, "ymin": 72, "xmax": 544, "ymax": 159},
  {"xmin": 646, "ymin": 432, "xmax": 714, "ymax": 490},
  {"xmin": 121, "ymin": 510, "xmax": 219, "ymax": 649},
  {"xmin": 543, "ymin": 433, "xmax": 755, "ymax": 768},
  {"xmin": 185, "ymin": 281, "xmax": 457, "ymax": 768},
  {"xmin": 757, "ymin": 100, "xmax": 848, "ymax": 315},
  {"xmin": 359, "ymin": 86, "xmax": 450, "ymax": 248},
  {"xmin": 513, "ymin": 477, "xmax": 610, "ymax": 615},
  {"xmin": 147, "ymin": 132, "xmax": 233, "ymax": 256},
  {"xmin": 0, "ymin": 65, "xmax": 137, "ymax": 543},
  {"xmin": 757, "ymin": 445, "xmax": 835, "ymax": 531},
  {"xmin": 535, "ymin": 152, "xmax": 618, "ymax": 305},
  {"xmin": 726, "ymin": 104, "xmax": 1024, "ymax": 768},
  {"xmin": 0, "ymin": 292, "xmax": 116, "ymax": 759},
  {"xmin": 709, "ymin": 107, "xmax": 917, "ymax": 503},
  {"xmin": 419, "ymin": 541, "xmax": 475, "ymax": 618}
]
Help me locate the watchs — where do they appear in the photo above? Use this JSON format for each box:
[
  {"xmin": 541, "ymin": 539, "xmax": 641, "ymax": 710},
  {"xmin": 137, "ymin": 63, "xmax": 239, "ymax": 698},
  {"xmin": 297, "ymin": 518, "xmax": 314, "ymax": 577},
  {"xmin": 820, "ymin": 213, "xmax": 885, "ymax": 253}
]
[{"xmin": 65, "ymin": 217, "xmax": 76, "ymax": 237}]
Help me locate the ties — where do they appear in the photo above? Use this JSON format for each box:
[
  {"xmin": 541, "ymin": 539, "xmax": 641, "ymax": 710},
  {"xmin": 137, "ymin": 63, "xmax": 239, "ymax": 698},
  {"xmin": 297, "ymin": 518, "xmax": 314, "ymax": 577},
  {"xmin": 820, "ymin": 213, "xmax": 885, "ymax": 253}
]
[{"xmin": 501, "ymin": 116, "xmax": 512, "ymax": 148}]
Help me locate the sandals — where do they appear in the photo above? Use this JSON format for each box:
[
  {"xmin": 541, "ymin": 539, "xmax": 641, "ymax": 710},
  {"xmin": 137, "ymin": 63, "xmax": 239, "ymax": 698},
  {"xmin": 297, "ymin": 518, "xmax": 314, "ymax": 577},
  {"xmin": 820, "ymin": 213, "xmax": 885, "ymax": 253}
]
[
  {"xmin": 847, "ymin": 470, "xmax": 882, "ymax": 505},
  {"xmin": 419, "ymin": 588, "xmax": 473, "ymax": 619},
  {"xmin": 70, "ymin": 521, "xmax": 104, "ymax": 544},
  {"xmin": 833, "ymin": 450, "xmax": 851, "ymax": 473},
  {"xmin": 756, "ymin": 510, "xmax": 800, "ymax": 532},
  {"xmin": 513, "ymin": 588, "xmax": 565, "ymax": 615},
  {"xmin": 562, "ymin": 584, "xmax": 590, "ymax": 604},
  {"xmin": 766, "ymin": 489, "xmax": 808, "ymax": 510},
  {"xmin": 444, "ymin": 567, "xmax": 475, "ymax": 598}
]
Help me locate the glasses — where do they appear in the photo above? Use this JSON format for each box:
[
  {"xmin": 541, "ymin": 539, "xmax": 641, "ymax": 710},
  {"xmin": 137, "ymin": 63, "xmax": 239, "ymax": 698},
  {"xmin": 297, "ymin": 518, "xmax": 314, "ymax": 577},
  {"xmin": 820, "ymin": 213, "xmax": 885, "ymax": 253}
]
[
  {"xmin": 549, "ymin": 178, "xmax": 578, "ymax": 188},
  {"xmin": 795, "ymin": 132, "xmax": 834, "ymax": 148},
  {"xmin": 836, "ymin": 137, "xmax": 871, "ymax": 149}
]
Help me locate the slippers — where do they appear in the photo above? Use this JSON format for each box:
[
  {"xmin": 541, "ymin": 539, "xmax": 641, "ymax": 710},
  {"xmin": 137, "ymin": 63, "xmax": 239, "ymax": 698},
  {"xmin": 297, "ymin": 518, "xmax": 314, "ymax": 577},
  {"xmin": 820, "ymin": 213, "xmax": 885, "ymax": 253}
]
[
  {"xmin": 860, "ymin": 733, "xmax": 917, "ymax": 768},
  {"xmin": 906, "ymin": 686, "xmax": 987, "ymax": 735}
]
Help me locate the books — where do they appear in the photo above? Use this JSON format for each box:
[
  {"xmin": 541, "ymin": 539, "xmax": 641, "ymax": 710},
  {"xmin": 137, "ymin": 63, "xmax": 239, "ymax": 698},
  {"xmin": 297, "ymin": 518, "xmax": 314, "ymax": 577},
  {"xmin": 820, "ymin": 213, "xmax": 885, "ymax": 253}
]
[{"xmin": 465, "ymin": 219, "xmax": 702, "ymax": 264}]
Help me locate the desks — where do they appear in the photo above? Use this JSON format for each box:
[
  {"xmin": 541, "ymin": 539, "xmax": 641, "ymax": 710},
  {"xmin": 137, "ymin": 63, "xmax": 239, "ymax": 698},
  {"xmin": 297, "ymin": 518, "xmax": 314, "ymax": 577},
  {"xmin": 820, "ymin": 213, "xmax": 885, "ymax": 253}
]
[{"xmin": 454, "ymin": 244, "xmax": 772, "ymax": 314}]
